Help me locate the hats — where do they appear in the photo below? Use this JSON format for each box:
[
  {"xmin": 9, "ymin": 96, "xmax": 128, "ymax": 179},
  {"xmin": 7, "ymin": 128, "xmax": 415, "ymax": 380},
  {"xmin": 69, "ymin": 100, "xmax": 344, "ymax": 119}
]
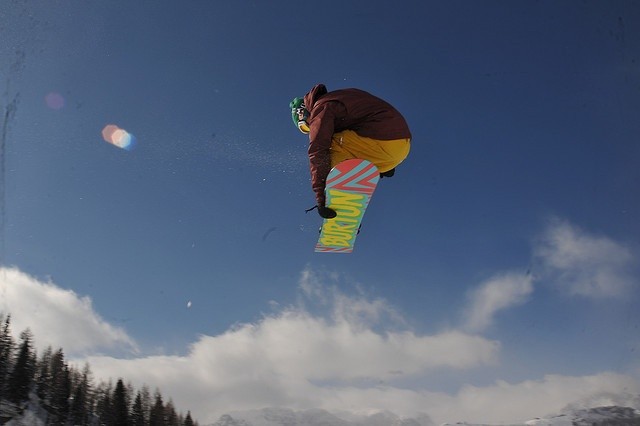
[{"xmin": 289, "ymin": 96, "xmax": 305, "ymax": 129}]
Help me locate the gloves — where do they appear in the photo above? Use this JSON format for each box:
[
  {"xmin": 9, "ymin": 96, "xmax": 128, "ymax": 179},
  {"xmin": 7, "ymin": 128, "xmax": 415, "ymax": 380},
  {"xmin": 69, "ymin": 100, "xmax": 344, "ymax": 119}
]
[{"xmin": 317, "ymin": 202, "xmax": 337, "ymax": 218}]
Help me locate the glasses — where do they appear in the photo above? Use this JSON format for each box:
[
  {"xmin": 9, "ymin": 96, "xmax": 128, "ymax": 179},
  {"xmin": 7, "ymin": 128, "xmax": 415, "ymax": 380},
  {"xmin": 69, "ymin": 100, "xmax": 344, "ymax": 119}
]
[{"xmin": 297, "ymin": 102, "xmax": 310, "ymax": 134}]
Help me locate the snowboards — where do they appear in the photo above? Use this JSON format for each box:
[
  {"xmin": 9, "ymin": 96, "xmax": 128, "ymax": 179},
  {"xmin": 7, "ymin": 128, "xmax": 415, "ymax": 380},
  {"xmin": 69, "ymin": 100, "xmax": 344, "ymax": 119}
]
[{"xmin": 313, "ymin": 157, "xmax": 380, "ymax": 255}]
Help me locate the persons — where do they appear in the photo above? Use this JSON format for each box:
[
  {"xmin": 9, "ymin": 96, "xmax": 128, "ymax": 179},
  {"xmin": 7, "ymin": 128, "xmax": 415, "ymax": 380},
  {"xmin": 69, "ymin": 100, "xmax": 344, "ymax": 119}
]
[{"xmin": 289, "ymin": 83, "xmax": 411, "ymax": 234}]
